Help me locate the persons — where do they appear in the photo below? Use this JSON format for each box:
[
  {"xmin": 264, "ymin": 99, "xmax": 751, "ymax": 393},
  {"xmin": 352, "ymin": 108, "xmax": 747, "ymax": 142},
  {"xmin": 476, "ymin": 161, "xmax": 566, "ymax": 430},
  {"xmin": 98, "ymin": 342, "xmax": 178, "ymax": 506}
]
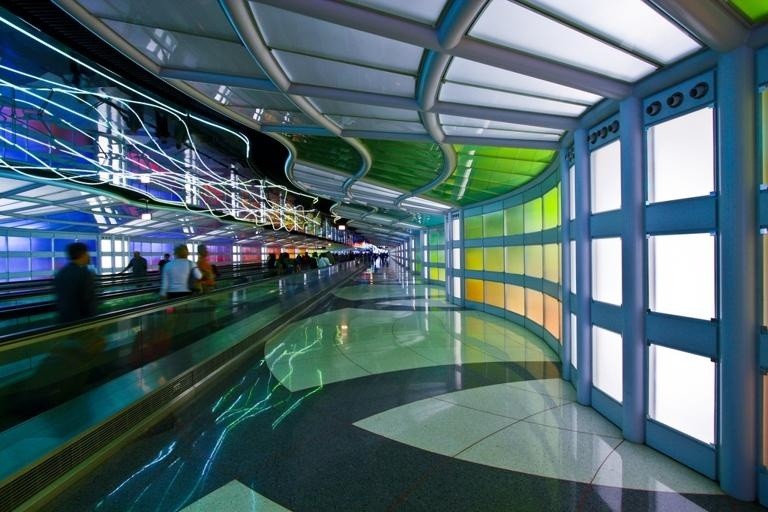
[
  {"xmin": 197, "ymin": 244, "xmax": 216, "ymax": 291},
  {"xmin": 157, "ymin": 253, "xmax": 171, "ymax": 272},
  {"xmin": 268, "ymin": 248, "xmax": 389, "ymax": 289},
  {"xmin": 49, "ymin": 242, "xmax": 103, "ymax": 325},
  {"xmin": 115, "ymin": 251, "xmax": 148, "ymax": 276},
  {"xmin": 159, "ymin": 244, "xmax": 202, "ymax": 300}
]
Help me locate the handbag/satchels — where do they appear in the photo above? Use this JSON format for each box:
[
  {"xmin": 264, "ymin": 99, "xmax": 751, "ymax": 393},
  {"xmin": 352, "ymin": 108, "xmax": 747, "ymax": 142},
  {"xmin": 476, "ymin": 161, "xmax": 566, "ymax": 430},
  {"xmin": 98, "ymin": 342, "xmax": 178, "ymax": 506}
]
[
  {"xmin": 202, "ymin": 264, "xmax": 218, "ymax": 281},
  {"xmin": 187, "ymin": 266, "xmax": 203, "ymax": 293}
]
